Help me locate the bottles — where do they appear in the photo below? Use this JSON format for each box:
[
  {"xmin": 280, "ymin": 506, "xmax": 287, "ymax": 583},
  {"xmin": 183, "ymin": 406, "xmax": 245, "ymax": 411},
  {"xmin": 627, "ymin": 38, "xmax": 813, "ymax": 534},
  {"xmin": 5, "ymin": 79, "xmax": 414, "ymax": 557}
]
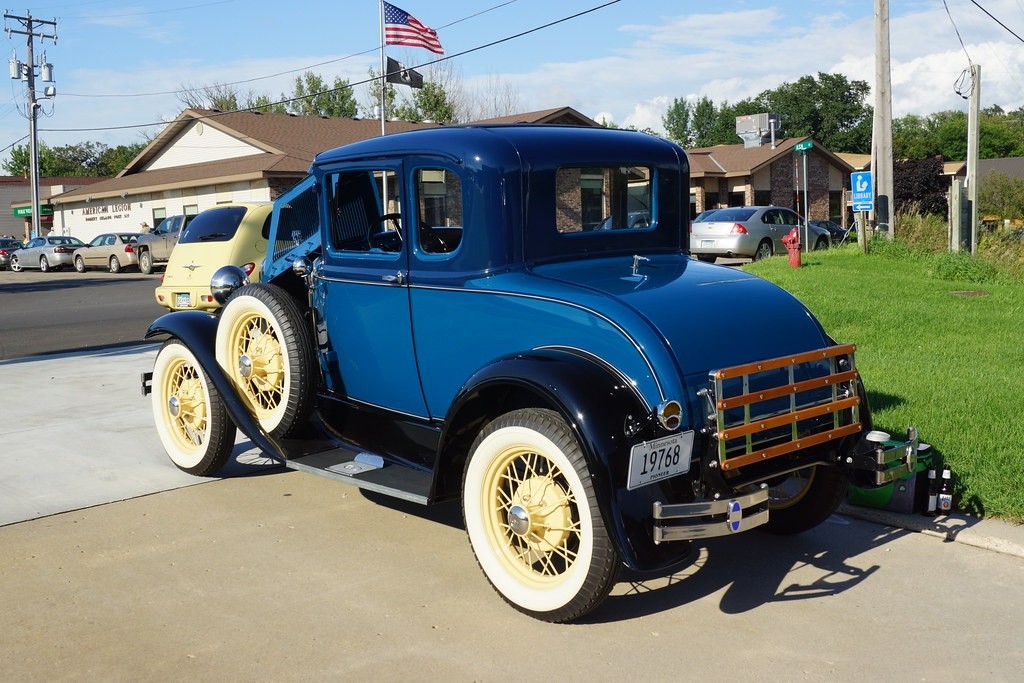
[{"xmin": 921, "ymin": 464, "xmax": 952, "ymax": 517}]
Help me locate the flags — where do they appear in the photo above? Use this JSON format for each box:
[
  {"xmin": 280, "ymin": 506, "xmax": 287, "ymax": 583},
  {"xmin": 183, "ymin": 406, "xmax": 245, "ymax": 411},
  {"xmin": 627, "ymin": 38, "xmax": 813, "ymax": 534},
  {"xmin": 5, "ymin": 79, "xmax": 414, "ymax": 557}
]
[
  {"xmin": 386, "ymin": 58, "xmax": 423, "ymax": 89},
  {"xmin": 383, "ymin": 1, "xmax": 444, "ymax": 55}
]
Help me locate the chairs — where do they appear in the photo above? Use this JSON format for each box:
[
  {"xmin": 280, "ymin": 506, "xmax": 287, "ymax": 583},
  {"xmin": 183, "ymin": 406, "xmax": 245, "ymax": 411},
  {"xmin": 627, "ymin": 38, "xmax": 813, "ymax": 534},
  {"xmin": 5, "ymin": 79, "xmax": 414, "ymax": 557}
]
[
  {"xmin": 108, "ymin": 238, "xmax": 137, "ymax": 245},
  {"xmin": 33, "ymin": 240, "xmax": 56, "ymax": 247}
]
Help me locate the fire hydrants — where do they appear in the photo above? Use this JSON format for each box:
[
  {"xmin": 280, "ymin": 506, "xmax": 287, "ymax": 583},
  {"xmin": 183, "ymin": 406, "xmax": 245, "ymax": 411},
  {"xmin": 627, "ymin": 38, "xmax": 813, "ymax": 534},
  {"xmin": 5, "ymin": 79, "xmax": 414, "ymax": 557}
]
[{"xmin": 782, "ymin": 229, "xmax": 802, "ymax": 269}]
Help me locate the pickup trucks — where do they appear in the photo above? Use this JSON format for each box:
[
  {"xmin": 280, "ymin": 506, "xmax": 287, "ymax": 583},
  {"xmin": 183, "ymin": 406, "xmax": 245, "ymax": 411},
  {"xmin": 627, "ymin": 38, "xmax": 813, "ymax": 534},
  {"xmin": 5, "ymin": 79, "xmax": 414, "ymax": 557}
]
[{"xmin": 136, "ymin": 213, "xmax": 200, "ymax": 274}]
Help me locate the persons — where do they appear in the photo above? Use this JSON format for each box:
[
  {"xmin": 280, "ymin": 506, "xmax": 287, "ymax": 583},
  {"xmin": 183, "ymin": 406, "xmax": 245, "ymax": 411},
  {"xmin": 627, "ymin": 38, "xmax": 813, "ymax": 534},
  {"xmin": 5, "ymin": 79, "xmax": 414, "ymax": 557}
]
[
  {"xmin": 140, "ymin": 222, "xmax": 150, "ymax": 234},
  {"xmin": 47, "ymin": 227, "xmax": 56, "ymax": 237},
  {"xmin": 22, "ymin": 234, "xmax": 28, "ymax": 244}
]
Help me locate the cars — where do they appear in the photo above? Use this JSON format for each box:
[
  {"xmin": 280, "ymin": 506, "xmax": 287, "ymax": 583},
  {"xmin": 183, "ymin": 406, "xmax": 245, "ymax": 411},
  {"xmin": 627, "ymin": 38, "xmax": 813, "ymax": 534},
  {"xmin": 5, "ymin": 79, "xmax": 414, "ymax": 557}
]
[
  {"xmin": 0, "ymin": 238, "xmax": 21, "ymax": 270},
  {"xmin": 72, "ymin": 232, "xmax": 139, "ymax": 273},
  {"xmin": 10, "ymin": 236, "xmax": 82, "ymax": 271},
  {"xmin": 154, "ymin": 201, "xmax": 297, "ymax": 314},
  {"xmin": 140, "ymin": 124, "xmax": 926, "ymax": 623},
  {"xmin": 810, "ymin": 219, "xmax": 850, "ymax": 244},
  {"xmin": 689, "ymin": 206, "xmax": 831, "ymax": 263}
]
